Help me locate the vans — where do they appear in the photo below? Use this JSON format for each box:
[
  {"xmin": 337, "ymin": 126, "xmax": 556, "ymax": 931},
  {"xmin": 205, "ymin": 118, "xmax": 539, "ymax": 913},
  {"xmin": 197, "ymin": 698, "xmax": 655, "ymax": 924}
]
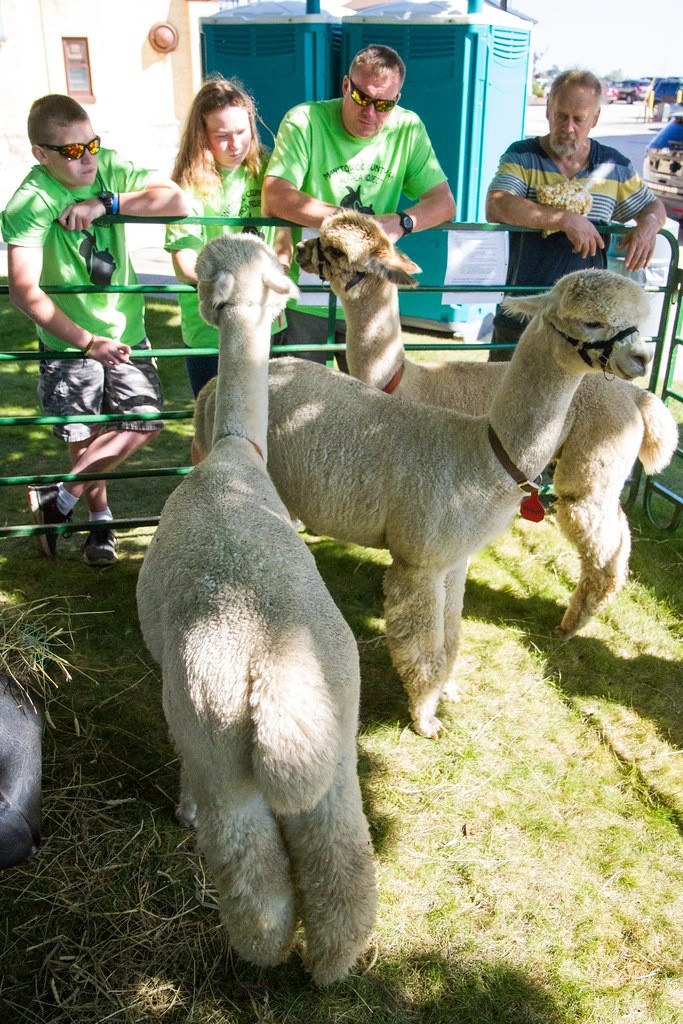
[{"xmin": 609, "ymin": 80, "xmax": 652, "ymax": 104}]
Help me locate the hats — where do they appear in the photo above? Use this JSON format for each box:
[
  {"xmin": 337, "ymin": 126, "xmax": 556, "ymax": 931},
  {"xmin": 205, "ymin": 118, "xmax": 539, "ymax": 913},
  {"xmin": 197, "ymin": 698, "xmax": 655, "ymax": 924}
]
[{"xmin": 149, "ymin": 22, "xmax": 179, "ymax": 53}]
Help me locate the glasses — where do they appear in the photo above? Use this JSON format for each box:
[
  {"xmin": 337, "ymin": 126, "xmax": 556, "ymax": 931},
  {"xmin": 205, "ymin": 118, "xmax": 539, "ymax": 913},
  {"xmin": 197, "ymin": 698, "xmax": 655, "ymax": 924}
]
[
  {"xmin": 37, "ymin": 135, "xmax": 100, "ymax": 160},
  {"xmin": 349, "ymin": 76, "xmax": 398, "ymax": 112}
]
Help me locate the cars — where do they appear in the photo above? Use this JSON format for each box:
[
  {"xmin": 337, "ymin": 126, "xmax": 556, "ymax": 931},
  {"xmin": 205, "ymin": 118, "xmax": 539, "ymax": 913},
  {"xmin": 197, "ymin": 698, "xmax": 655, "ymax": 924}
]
[
  {"xmin": 641, "ymin": 112, "xmax": 683, "ymax": 229},
  {"xmin": 599, "ymin": 81, "xmax": 619, "ymax": 104}
]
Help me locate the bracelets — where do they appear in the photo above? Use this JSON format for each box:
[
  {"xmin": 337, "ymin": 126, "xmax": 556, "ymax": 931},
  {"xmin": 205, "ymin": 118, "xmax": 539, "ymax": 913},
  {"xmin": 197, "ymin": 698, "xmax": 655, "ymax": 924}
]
[
  {"xmin": 111, "ymin": 192, "xmax": 118, "ymax": 215},
  {"xmin": 117, "ymin": 191, "xmax": 120, "ymax": 214},
  {"xmin": 84, "ymin": 334, "xmax": 95, "ymax": 357}
]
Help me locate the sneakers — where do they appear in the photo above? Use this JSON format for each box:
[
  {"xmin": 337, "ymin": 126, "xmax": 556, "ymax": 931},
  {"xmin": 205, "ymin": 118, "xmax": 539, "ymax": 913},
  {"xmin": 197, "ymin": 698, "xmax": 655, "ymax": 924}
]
[
  {"xmin": 83, "ymin": 520, "xmax": 119, "ymax": 565},
  {"xmin": 28, "ymin": 485, "xmax": 74, "ymax": 557}
]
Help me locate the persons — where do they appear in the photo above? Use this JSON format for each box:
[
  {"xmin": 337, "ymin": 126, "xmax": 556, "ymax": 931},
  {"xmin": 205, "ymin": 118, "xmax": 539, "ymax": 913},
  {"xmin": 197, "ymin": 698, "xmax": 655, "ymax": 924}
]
[
  {"xmin": 0, "ymin": 93, "xmax": 191, "ymax": 565},
  {"xmin": 485, "ymin": 69, "xmax": 666, "ymax": 363},
  {"xmin": 258, "ymin": 41, "xmax": 457, "ymax": 375},
  {"xmin": 162, "ymin": 68, "xmax": 295, "ymax": 401}
]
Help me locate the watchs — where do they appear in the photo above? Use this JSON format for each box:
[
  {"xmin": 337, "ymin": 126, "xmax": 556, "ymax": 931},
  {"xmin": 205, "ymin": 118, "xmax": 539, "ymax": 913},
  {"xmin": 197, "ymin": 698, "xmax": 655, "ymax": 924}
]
[
  {"xmin": 96, "ymin": 190, "xmax": 114, "ymax": 214},
  {"xmin": 394, "ymin": 210, "xmax": 413, "ymax": 235}
]
